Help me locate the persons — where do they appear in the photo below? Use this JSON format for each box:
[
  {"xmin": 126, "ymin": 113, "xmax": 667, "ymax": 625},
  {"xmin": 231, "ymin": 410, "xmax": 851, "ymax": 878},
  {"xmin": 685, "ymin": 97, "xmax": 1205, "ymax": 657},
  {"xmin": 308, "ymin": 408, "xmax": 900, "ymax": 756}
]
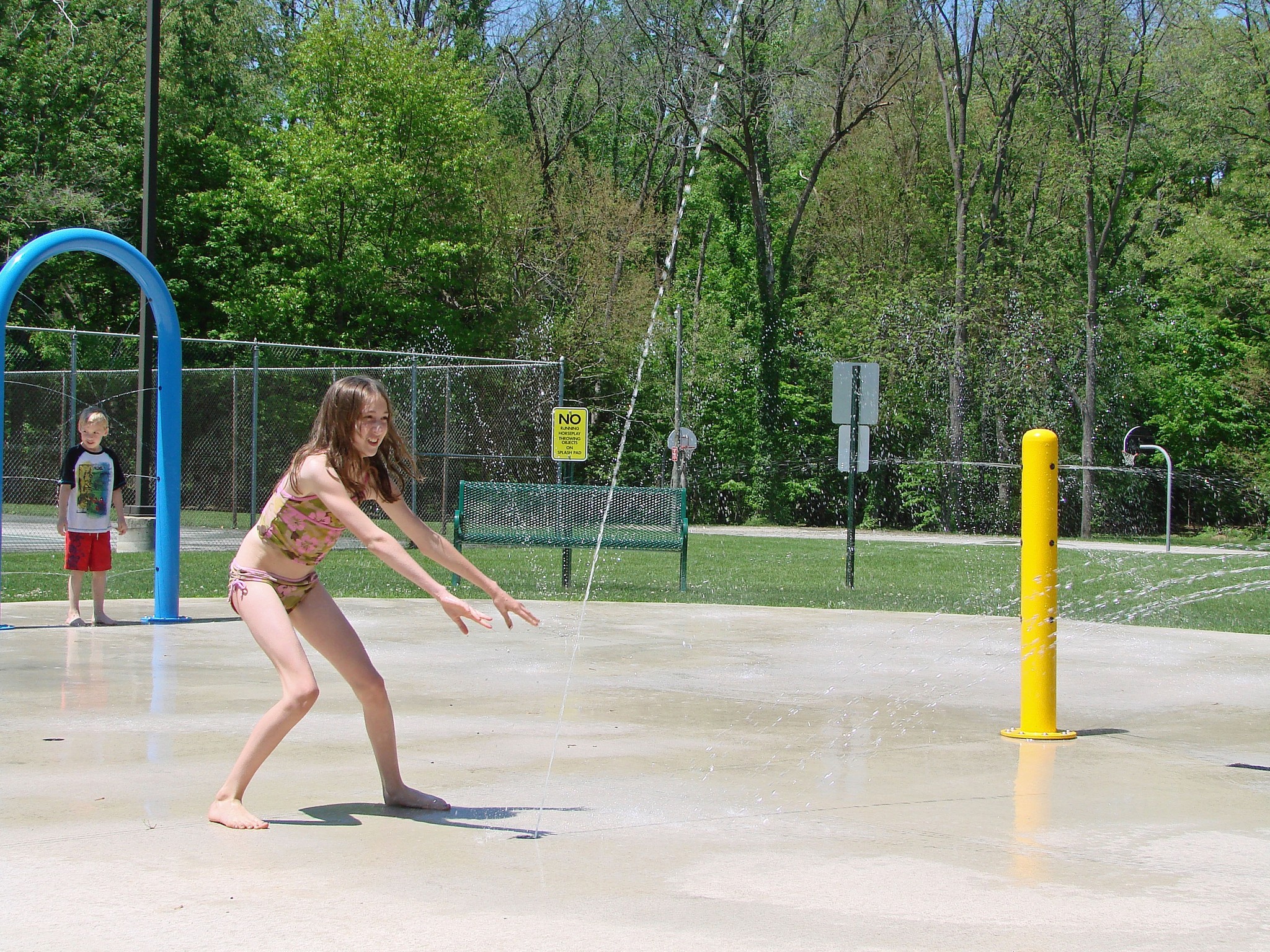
[
  {"xmin": 55, "ymin": 407, "xmax": 128, "ymax": 629},
  {"xmin": 206, "ymin": 371, "xmax": 540, "ymax": 829}
]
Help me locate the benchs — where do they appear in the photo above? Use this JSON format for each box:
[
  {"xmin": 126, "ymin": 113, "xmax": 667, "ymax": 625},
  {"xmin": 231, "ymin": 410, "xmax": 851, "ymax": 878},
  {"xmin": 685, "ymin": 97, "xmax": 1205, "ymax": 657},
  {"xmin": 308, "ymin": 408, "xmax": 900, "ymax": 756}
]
[{"xmin": 452, "ymin": 480, "xmax": 688, "ymax": 594}]
